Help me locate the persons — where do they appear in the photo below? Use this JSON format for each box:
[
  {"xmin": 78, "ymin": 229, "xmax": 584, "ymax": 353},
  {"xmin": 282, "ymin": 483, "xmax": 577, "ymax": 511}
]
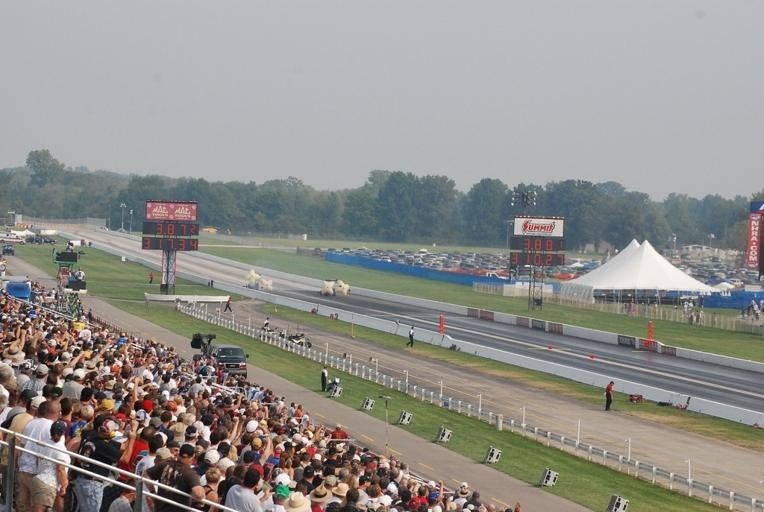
[
  {"xmin": 683, "ymin": 300, "xmax": 695, "ymax": 315},
  {"xmin": 750, "ymin": 298, "xmax": 764, "ymax": 320},
  {"xmin": 604, "ymin": 381, "xmax": 614, "ymax": 411}
]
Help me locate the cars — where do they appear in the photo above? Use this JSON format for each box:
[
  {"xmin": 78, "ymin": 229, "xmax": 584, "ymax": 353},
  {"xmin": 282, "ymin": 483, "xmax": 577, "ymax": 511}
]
[
  {"xmin": 317, "ymin": 244, "xmax": 599, "ymax": 284},
  {"xmin": 35, "ymin": 233, "xmax": 56, "ymax": 246},
  {"xmin": 669, "ymin": 253, "xmax": 763, "ymax": 291}
]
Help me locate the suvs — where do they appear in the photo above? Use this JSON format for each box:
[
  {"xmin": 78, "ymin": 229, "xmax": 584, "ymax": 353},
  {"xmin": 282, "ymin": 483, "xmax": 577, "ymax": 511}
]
[{"xmin": 3, "ymin": 245, "xmax": 15, "ymax": 256}]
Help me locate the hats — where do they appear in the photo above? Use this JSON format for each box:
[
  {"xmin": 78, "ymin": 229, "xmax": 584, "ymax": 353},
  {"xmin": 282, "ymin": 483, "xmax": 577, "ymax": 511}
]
[
  {"xmin": 0, "ymin": 314, "xmax": 148, "ymax": 439},
  {"xmin": 237, "ymin": 376, "xmax": 488, "ymax": 512},
  {"xmin": 148, "ymin": 341, "xmax": 237, "ymax": 465}
]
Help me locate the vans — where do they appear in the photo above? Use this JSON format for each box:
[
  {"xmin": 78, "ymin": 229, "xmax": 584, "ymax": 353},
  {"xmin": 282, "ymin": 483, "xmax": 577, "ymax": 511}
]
[{"xmin": 213, "ymin": 344, "xmax": 249, "ymax": 379}]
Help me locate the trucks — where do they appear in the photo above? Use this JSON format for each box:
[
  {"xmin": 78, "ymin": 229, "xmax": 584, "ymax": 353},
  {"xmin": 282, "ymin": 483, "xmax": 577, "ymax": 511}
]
[{"xmin": 0, "ymin": 233, "xmax": 26, "ymax": 246}]
